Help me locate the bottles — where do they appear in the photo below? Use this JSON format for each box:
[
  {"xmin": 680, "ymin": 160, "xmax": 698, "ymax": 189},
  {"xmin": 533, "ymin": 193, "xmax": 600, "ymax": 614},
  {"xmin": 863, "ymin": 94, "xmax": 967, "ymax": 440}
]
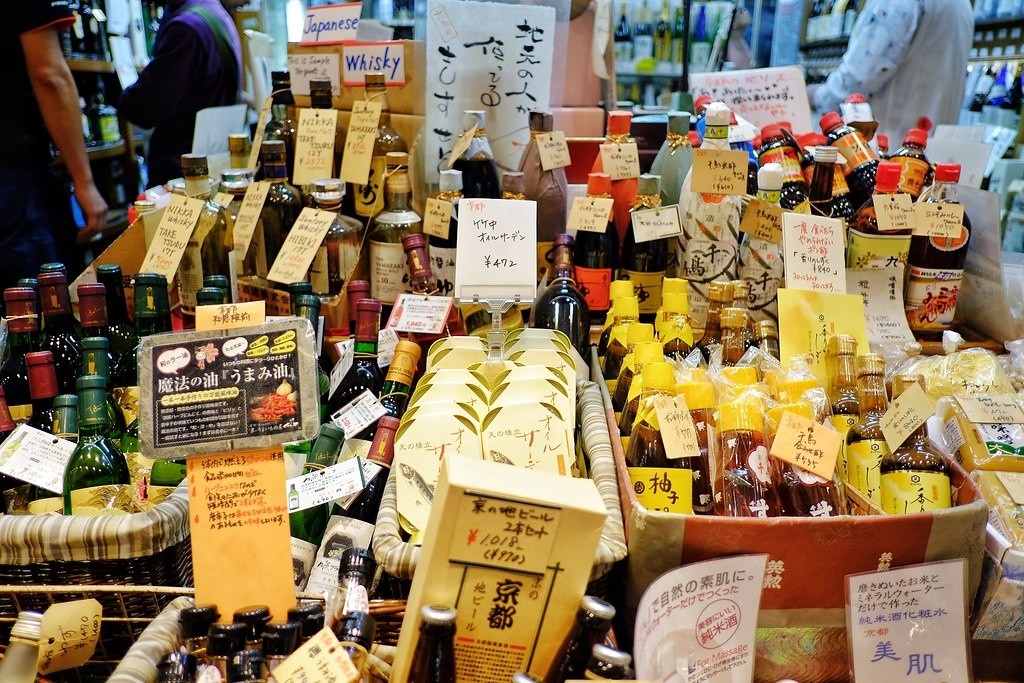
[
  {"xmin": 400, "ymin": 110, "xmax": 567, "ymax": 364},
  {"xmin": 134, "ymin": 201, "xmax": 156, "ymax": 217},
  {"xmin": 648, "ymin": 110, "xmax": 693, "ymax": 212},
  {"xmin": 282, "ymin": 280, "xmax": 422, "ymax": 630},
  {"xmin": 0, "ymin": 263, "xmax": 188, "ymax": 514},
  {"xmin": 572, "ymin": 173, "xmax": 616, "ymax": 325},
  {"xmin": 539, "ymin": 596, "xmax": 632, "ymax": 682},
  {"xmin": 618, "ymin": 174, "xmax": 667, "ymax": 324},
  {"xmin": 528, "ymin": 233, "xmax": 589, "ymax": 365},
  {"xmin": 597, "ymin": 277, "xmax": 841, "ymax": 519},
  {"xmin": 406, "ymin": 604, "xmax": 457, "ymax": 683},
  {"xmin": 175, "ymin": 71, "xmax": 424, "ymax": 337},
  {"xmin": 800, "ymin": 0, "xmax": 1024, "ymax": 160},
  {"xmin": 155, "ymin": 603, "xmax": 324, "ymax": 683},
  {"xmin": 677, "ymin": 92, "xmax": 970, "ymax": 332},
  {"xmin": 58, "ymin": 0, "xmax": 128, "ymax": 228},
  {"xmin": 589, "ymin": 110, "xmax": 638, "ymax": 248},
  {"xmin": 324, "ymin": 547, "xmax": 377, "ymax": 655},
  {"xmin": 827, "ymin": 335, "xmax": 952, "ymax": 514},
  {"xmin": 142, "ymin": 1, "xmax": 160, "ymax": 56},
  {"xmin": 0, "ymin": 611, "xmax": 44, "ymax": 683},
  {"xmin": 614, "ymin": 0, "xmax": 713, "ymax": 106}
]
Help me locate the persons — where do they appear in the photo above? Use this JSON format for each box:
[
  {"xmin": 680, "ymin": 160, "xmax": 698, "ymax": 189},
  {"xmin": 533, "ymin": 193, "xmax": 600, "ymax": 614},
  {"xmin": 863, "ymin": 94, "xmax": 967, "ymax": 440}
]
[
  {"xmin": 119, "ymin": 0, "xmax": 243, "ymax": 190},
  {"xmin": 0, "ymin": 0, "xmax": 108, "ymax": 320},
  {"xmin": 806, "ymin": 0, "xmax": 975, "ymax": 158},
  {"xmin": 508, "ymin": 0, "xmax": 603, "ymax": 108},
  {"xmin": 727, "ymin": 7, "xmax": 751, "ymax": 68}
]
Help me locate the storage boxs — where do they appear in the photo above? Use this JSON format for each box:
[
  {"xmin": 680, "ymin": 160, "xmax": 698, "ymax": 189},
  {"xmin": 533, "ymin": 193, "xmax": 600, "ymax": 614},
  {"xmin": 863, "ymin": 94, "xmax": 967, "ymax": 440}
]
[{"xmin": 0, "ymin": 40, "xmax": 1024, "ymax": 683}]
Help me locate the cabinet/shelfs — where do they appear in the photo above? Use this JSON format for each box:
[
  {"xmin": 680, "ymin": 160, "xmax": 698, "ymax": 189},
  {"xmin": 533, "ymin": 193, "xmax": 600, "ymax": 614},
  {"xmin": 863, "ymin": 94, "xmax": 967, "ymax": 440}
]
[{"xmin": 50, "ymin": 0, "xmax": 143, "ymax": 237}]
